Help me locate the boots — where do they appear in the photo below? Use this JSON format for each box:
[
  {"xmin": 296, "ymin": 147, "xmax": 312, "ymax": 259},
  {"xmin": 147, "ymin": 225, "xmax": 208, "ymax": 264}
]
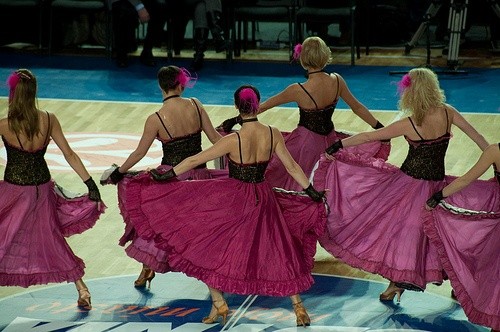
[
  {"xmin": 188, "ymin": 27, "xmax": 210, "ymax": 71},
  {"xmin": 205, "ymin": 9, "xmax": 239, "ymax": 53}
]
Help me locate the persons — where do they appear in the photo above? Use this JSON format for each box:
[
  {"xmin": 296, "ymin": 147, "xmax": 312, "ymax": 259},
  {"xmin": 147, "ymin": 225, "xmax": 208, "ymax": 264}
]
[
  {"xmin": 100, "ymin": 38, "xmax": 500, "ymax": 332},
  {"xmin": 108, "ymin": 0, "xmax": 165, "ymax": 68},
  {"xmin": 190, "ymin": 0, "xmax": 228, "ymax": 70},
  {"xmin": 0, "ymin": 68, "xmax": 108, "ymax": 310}
]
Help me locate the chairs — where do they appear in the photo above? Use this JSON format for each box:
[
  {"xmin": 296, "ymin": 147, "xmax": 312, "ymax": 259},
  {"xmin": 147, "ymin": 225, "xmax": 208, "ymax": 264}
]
[{"xmin": 0, "ymin": 0, "xmax": 360, "ymax": 65}]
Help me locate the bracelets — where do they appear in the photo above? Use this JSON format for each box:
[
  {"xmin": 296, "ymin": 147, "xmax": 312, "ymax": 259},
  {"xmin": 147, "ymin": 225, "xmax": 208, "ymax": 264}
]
[{"xmin": 135, "ymin": 4, "xmax": 144, "ymax": 11}]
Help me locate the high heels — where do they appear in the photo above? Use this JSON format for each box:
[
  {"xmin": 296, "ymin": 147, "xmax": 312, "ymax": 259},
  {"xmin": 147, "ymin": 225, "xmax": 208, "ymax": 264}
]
[
  {"xmin": 134, "ymin": 267, "xmax": 155, "ymax": 288},
  {"xmin": 380, "ymin": 281, "xmax": 404, "ymax": 302},
  {"xmin": 292, "ymin": 301, "xmax": 311, "ymax": 328},
  {"xmin": 78, "ymin": 288, "xmax": 92, "ymax": 308},
  {"xmin": 202, "ymin": 298, "xmax": 229, "ymax": 326}
]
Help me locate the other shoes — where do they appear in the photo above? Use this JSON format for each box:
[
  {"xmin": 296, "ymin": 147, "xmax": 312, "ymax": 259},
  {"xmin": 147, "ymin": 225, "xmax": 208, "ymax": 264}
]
[
  {"xmin": 119, "ymin": 53, "xmax": 129, "ymax": 68},
  {"xmin": 139, "ymin": 50, "xmax": 157, "ymax": 68}
]
